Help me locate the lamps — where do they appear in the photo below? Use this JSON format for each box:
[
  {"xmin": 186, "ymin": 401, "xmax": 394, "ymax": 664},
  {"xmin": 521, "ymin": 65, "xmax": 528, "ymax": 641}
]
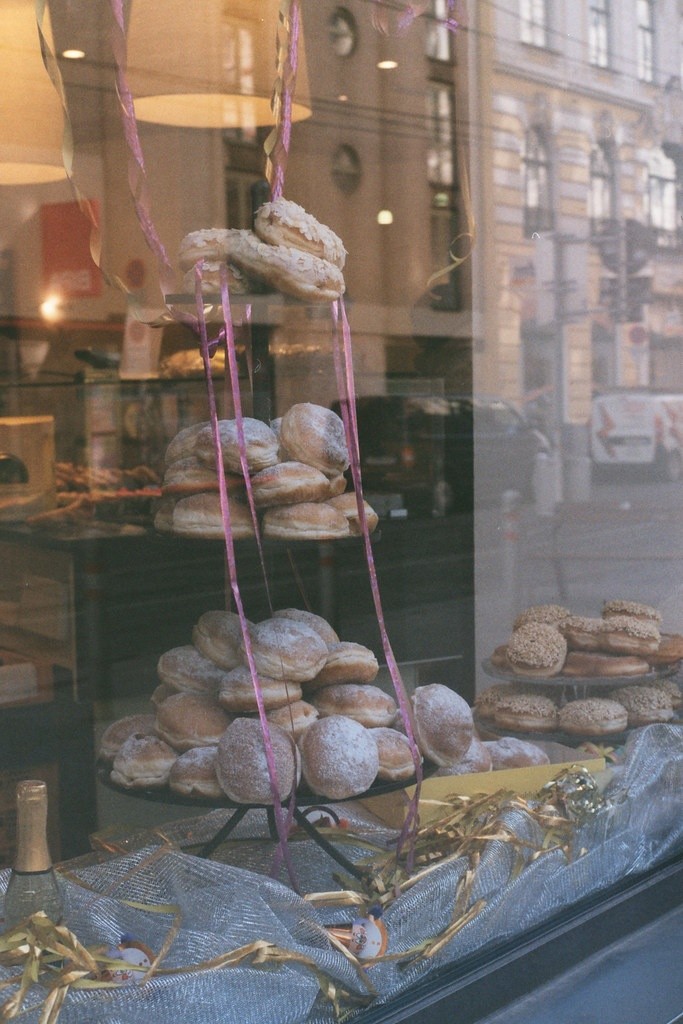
[{"xmin": 119, "ymin": 1, "xmax": 312, "ymax": 127}]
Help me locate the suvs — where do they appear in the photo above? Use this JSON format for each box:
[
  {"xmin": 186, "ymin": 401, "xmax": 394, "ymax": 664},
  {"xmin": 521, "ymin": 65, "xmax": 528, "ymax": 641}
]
[{"xmin": 319, "ymin": 394, "xmax": 556, "ymax": 520}]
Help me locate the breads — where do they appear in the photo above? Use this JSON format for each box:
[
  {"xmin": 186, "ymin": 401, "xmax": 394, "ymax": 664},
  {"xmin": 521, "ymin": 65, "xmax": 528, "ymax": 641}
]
[
  {"xmin": 474, "ymin": 595, "xmax": 683, "ymax": 737},
  {"xmin": 98, "ymin": 606, "xmax": 552, "ymax": 808},
  {"xmin": 162, "ymin": 404, "xmax": 378, "ymax": 542},
  {"xmin": 174, "ymin": 197, "xmax": 346, "ymax": 304},
  {"xmin": 53, "ymin": 462, "xmax": 155, "ymax": 494}
]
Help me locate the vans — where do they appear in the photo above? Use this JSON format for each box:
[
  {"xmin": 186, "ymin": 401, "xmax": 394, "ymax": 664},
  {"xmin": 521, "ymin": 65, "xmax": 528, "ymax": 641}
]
[
  {"xmin": 588, "ymin": 390, "xmax": 683, "ymax": 477},
  {"xmin": 0, "ymin": 449, "xmax": 44, "ymax": 527}
]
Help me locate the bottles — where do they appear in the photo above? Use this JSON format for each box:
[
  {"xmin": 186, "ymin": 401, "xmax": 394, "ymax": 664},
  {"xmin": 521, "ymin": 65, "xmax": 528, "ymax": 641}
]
[{"xmin": 4, "ymin": 781, "xmax": 62, "ymax": 936}]
